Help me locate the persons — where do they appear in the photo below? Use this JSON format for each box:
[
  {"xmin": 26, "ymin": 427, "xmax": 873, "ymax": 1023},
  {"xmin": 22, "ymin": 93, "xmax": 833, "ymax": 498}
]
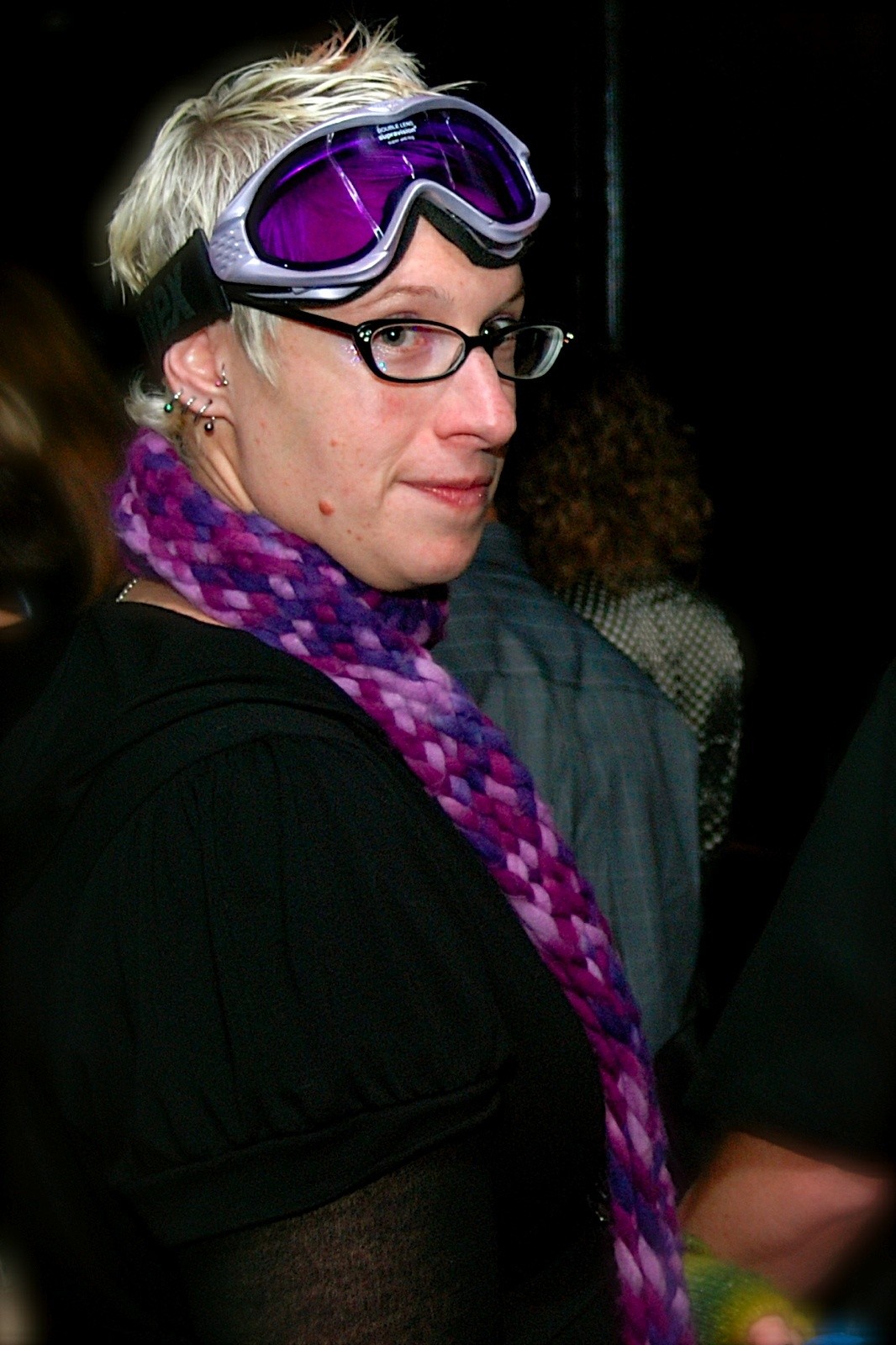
[
  {"xmin": 0, "ymin": 24, "xmax": 700, "ymax": 1344},
  {"xmin": 426, "ymin": 357, "xmax": 896, "ymax": 1345},
  {"xmin": 1, "ymin": 264, "xmax": 140, "ymax": 735}
]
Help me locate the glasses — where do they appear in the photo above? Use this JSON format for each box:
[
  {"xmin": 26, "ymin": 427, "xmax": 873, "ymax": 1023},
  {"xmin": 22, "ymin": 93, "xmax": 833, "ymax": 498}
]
[
  {"xmin": 112, "ymin": 92, "xmax": 551, "ymax": 357},
  {"xmin": 214, "ymin": 267, "xmax": 572, "ymax": 383}
]
